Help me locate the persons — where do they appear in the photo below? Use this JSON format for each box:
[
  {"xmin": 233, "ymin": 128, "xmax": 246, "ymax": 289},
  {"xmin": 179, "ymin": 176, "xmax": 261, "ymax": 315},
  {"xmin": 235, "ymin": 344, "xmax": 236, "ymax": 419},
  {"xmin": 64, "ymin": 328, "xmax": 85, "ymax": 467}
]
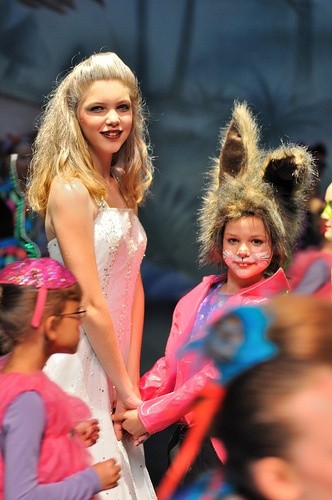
[
  {"xmin": 112, "ymin": 98, "xmax": 315, "ymax": 489},
  {"xmin": 155, "ymin": 142, "xmax": 332, "ymax": 500},
  {"xmin": 27, "ymin": 51, "xmax": 158, "ymax": 500},
  {"xmin": 0, "ymin": 258, "xmax": 122, "ymax": 500},
  {"xmin": 0, "ymin": 132, "xmax": 50, "ymax": 270}
]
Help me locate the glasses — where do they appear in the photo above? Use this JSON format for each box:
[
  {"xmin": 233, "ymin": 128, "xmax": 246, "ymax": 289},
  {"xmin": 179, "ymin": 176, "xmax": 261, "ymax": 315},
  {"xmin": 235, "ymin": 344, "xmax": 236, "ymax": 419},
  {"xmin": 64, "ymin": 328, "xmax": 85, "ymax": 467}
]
[{"xmin": 59, "ymin": 306, "xmax": 87, "ymax": 318}]
